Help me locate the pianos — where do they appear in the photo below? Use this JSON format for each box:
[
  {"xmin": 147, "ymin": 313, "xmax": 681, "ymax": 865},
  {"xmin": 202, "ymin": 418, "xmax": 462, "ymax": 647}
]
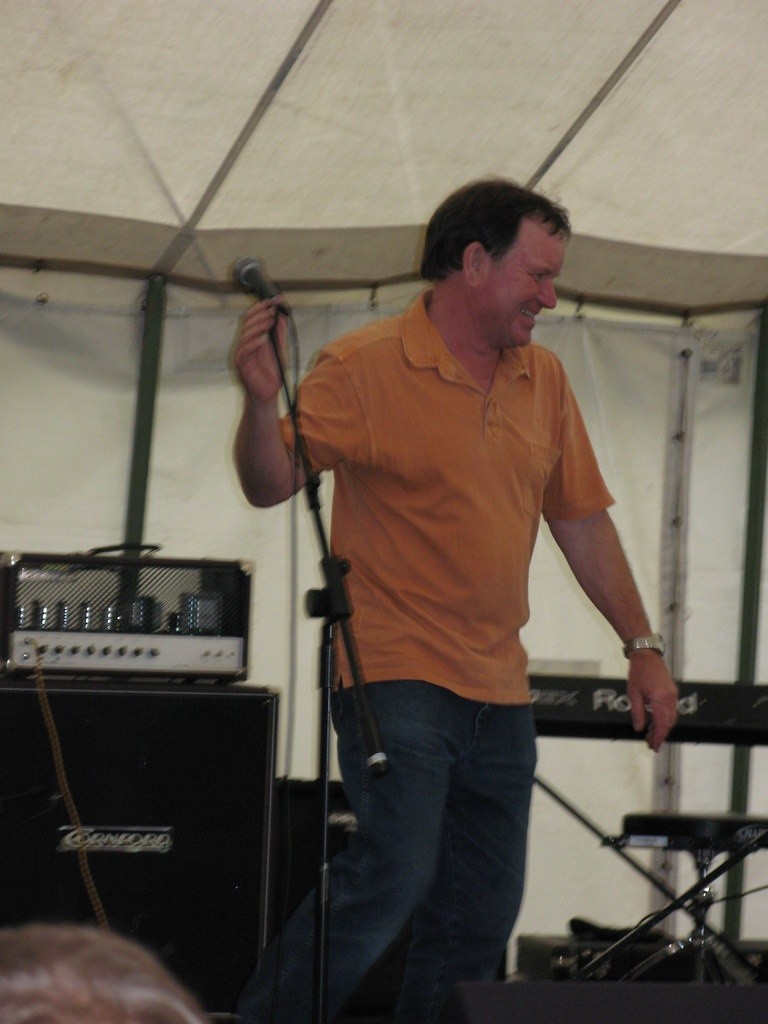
[{"xmin": 524, "ymin": 673, "xmax": 768, "ymax": 749}]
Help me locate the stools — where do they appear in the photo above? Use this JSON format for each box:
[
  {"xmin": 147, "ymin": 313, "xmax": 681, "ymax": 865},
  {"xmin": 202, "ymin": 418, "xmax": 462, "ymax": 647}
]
[{"xmin": 601, "ymin": 814, "xmax": 768, "ymax": 984}]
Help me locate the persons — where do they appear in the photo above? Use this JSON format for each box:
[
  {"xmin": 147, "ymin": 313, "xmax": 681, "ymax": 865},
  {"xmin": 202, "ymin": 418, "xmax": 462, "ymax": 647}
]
[{"xmin": 232, "ymin": 180, "xmax": 678, "ymax": 1022}]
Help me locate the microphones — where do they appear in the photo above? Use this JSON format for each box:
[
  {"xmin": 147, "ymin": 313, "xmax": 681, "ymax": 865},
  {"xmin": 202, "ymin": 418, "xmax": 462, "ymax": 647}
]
[{"xmin": 234, "ymin": 255, "xmax": 292, "ymax": 317}]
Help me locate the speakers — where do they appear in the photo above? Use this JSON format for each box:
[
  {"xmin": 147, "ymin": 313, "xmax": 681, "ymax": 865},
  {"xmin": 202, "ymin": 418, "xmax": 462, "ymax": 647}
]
[
  {"xmin": 0, "ymin": 677, "xmax": 282, "ymax": 1024},
  {"xmin": 422, "ymin": 981, "xmax": 768, "ymax": 1024}
]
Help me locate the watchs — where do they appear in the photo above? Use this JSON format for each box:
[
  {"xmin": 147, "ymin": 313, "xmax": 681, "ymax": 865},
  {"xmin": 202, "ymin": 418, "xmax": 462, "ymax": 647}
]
[{"xmin": 623, "ymin": 633, "xmax": 666, "ymax": 660}]
